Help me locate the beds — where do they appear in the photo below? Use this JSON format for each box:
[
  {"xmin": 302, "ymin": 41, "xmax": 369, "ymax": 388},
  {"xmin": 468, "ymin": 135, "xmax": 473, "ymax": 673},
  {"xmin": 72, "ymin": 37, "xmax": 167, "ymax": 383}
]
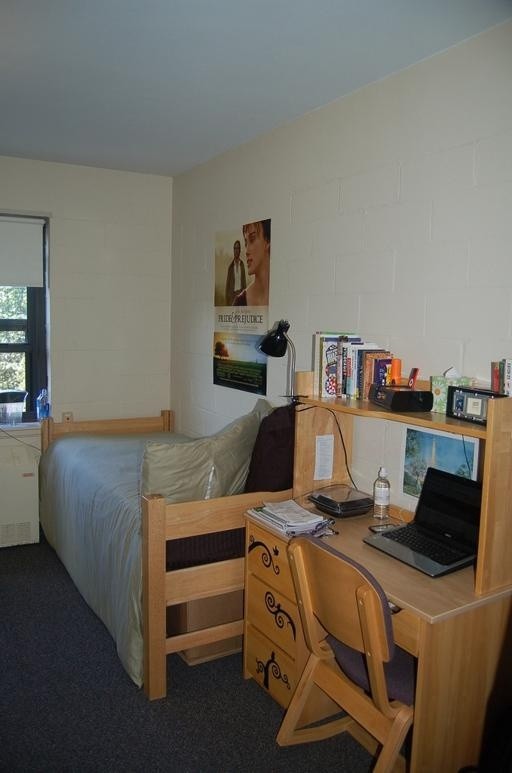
[{"xmin": 40, "ymin": 410, "xmax": 293, "ymax": 701}]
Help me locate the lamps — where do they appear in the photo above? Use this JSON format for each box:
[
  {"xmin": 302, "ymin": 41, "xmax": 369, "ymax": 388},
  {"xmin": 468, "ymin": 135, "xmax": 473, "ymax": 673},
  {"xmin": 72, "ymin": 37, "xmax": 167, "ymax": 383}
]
[{"xmin": 259, "ymin": 320, "xmax": 308, "ymax": 408}]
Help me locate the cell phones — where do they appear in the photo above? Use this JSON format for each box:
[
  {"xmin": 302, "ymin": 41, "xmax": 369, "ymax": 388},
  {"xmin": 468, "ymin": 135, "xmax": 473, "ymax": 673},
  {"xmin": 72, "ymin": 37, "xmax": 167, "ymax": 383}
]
[{"xmin": 368, "ymin": 524, "xmax": 396, "ymax": 533}]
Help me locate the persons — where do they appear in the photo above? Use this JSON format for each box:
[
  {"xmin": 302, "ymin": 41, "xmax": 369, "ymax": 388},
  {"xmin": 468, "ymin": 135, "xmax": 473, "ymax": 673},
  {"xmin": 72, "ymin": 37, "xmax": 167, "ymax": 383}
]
[
  {"xmin": 231, "ymin": 220, "xmax": 269, "ymax": 306},
  {"xmin": 224, "ymin": 240, "xmax": 246, "ymax": 307}
]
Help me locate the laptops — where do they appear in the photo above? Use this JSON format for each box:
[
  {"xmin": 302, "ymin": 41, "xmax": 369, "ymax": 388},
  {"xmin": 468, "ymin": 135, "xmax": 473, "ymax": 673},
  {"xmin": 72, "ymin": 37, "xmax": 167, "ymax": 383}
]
[{"xmin": 363, "ymin": 467, "xmax": 483, "ymax": 578}]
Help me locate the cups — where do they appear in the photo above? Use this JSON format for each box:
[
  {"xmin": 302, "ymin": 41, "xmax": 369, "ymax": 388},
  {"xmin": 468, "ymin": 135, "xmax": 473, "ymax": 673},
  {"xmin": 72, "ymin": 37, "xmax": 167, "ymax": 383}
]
[{"xmin": 373, "ymin": 466, "xmax": 391, "ymax": 521}]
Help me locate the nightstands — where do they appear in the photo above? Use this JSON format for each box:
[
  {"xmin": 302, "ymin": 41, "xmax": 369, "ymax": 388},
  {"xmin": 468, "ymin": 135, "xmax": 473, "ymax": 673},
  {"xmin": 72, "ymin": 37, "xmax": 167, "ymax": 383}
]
[{"xmin": 0, "ymin": 422, "xmax": 41, "ymax": 548}]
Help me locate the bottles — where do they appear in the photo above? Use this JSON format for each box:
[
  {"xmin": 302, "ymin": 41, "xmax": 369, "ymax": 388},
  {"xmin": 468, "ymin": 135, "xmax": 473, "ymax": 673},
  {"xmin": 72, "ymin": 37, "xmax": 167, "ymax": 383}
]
[{"xmin": 37, "ymin": 389, "xmax": 49, "ymax": 422}]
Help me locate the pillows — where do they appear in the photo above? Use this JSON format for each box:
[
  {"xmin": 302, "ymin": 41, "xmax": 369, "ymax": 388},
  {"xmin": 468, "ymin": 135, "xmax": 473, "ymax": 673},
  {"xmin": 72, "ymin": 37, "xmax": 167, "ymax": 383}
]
[{"xmin": 139, "ymin": 398, "xmax": 293, "ymax": 537}]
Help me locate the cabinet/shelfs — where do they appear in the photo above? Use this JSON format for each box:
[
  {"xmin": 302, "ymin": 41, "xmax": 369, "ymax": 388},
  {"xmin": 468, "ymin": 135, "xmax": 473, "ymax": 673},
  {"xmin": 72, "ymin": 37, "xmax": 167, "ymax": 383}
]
[{"xmin": 242, "ymin": 371, "xmax": 512, "ymax": 773}]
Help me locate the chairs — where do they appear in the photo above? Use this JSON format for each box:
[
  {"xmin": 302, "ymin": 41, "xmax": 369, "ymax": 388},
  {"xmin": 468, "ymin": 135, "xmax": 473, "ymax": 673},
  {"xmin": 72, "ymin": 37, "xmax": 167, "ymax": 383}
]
[{"xmin": 275, "ymin": 534, "xmax": 413, "ymax": 773}]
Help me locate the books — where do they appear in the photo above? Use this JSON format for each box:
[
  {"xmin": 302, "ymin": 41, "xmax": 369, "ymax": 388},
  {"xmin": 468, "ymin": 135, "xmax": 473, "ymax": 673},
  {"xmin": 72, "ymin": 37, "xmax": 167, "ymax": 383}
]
[
  {"xmin": 249, "ymin": 497, "xmax": 326, "ymax": 535},
  {"xmin": 309, "ymin": 331, "xmax": 394, "ymax": 403},
  {"xmin": 490, "ymin": 358, "xmax": 512, "ymax": 396}
]
